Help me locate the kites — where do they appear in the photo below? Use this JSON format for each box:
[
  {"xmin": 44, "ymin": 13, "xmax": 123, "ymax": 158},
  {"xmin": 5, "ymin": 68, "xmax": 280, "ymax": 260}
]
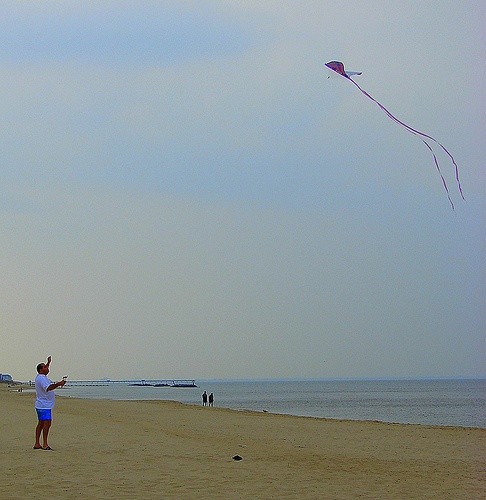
[{"xmin": 324, "ymin": 60, "xmax": 465, "ymax": 210}]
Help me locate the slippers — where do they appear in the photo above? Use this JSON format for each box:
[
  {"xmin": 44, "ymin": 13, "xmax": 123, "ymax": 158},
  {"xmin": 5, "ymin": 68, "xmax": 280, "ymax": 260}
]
[
  {"xmin": 42, "ymin": 446, "xmax": 52, "ymax": 450},
  {"xmin": 34, "ymin": 446, "xmax": 42, "ymax": 449}
]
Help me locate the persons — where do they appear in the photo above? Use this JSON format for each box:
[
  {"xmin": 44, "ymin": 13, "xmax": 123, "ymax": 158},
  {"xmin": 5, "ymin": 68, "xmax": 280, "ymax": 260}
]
[
  {"xmin": 33, "ymin": 356, "xmax": 67, "ymax": 451},
  {"xmin": 202, "ymin": 391, "xmax": 207, "ymax": 407},
  {"xmin": 209, "ymin": 393, "xmax": 213, "ymax": 407}
]
